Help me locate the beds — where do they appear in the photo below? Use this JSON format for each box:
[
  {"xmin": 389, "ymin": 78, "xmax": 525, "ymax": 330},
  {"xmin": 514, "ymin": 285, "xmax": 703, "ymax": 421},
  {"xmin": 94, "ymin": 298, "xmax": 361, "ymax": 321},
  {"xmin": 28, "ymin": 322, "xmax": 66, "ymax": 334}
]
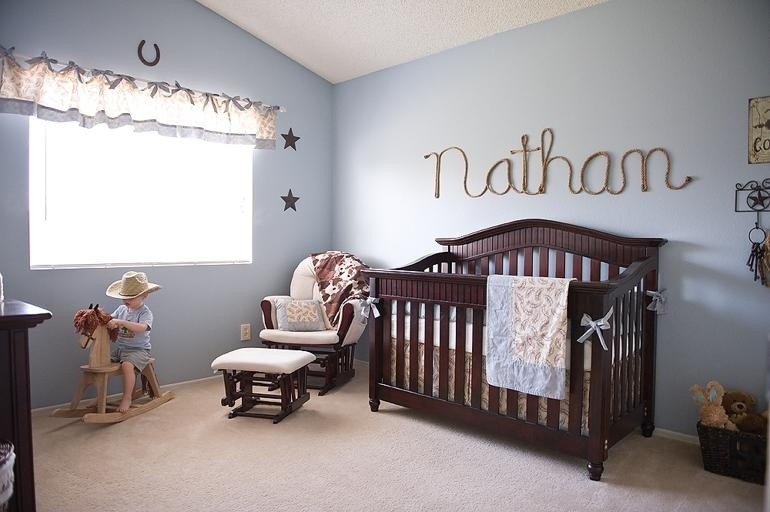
[{"xmin": 361, "ymin": 217, "xmax": 668, "ymax": 482}]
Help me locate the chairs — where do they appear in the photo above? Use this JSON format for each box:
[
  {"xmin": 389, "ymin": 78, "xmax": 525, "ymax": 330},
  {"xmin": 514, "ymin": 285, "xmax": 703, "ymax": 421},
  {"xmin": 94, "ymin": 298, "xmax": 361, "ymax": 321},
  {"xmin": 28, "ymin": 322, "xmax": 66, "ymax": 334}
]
[{"xmin": 260, "ymin": 250, "xmax": 377, "ymax": 397}]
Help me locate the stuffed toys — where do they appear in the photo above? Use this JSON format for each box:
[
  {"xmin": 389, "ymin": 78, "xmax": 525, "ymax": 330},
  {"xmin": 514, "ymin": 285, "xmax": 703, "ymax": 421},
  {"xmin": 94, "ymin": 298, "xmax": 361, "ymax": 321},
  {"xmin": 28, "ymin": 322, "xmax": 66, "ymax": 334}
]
[
  {"xmin": 721, "ymin": 390, "xmax": 766, "ymax": 434},
  {"xmin": 687, "ymin": 380, "xmax": 742, "ymax": 432}
]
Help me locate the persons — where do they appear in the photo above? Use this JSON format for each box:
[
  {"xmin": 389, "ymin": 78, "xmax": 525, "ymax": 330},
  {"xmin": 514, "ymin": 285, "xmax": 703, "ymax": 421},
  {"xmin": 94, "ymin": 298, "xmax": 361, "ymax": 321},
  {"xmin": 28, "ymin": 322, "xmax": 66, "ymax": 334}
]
[{"xmin": 84, "ymin": 270, "xmax": 155, "ymax": 413}]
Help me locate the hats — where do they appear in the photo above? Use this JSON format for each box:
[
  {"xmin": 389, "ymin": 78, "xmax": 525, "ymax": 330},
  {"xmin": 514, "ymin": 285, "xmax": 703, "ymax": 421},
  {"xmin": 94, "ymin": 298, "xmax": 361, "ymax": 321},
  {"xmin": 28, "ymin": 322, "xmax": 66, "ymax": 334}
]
[{"xmin": 106, "ymin": 271, "xmax": 162, "ymax": 299}]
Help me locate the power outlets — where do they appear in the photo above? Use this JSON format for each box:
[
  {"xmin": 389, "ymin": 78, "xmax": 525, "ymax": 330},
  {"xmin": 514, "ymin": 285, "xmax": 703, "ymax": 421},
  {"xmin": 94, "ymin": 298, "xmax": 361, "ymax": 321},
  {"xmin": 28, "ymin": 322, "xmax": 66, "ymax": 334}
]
[{"xmin": 240, "ymin": 324, "xmax": 251, "ymax": 340}]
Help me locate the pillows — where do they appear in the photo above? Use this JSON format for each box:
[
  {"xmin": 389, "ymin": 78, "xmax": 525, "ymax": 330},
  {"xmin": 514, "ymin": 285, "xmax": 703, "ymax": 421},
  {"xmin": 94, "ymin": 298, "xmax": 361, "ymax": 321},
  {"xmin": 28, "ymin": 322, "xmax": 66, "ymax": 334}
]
[
  {"xmin": 384, "ymin": 294, "xmax": 458, "ymax": 322},
  {"xmin": 273, "ymin": 295, "xmax": 325, "ymax": 333}
]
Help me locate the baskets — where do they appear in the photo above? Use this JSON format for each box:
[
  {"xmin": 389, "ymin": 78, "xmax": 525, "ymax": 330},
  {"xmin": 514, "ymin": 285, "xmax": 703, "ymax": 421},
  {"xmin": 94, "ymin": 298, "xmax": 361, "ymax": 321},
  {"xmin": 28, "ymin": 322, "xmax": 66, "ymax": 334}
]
[{"xmin": 697, "ymin": 419, "xmax": 766, "ymax": 485}]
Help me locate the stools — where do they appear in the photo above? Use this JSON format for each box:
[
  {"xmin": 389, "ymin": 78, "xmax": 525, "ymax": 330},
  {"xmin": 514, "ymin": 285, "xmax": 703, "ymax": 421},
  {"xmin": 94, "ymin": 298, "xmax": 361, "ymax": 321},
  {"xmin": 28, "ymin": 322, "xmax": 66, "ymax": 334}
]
[{"xmin": 210, "ymin": 345, "xmax": 318, "ymax": 425}]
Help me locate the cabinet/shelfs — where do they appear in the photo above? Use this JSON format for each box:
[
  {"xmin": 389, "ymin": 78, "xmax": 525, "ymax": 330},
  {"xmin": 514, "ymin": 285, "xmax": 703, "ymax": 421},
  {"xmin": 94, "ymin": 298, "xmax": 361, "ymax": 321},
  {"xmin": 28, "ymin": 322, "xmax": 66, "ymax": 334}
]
[{"xmin": 0, "ymin": 299, "xmax": 54, "ymax": 512}]
